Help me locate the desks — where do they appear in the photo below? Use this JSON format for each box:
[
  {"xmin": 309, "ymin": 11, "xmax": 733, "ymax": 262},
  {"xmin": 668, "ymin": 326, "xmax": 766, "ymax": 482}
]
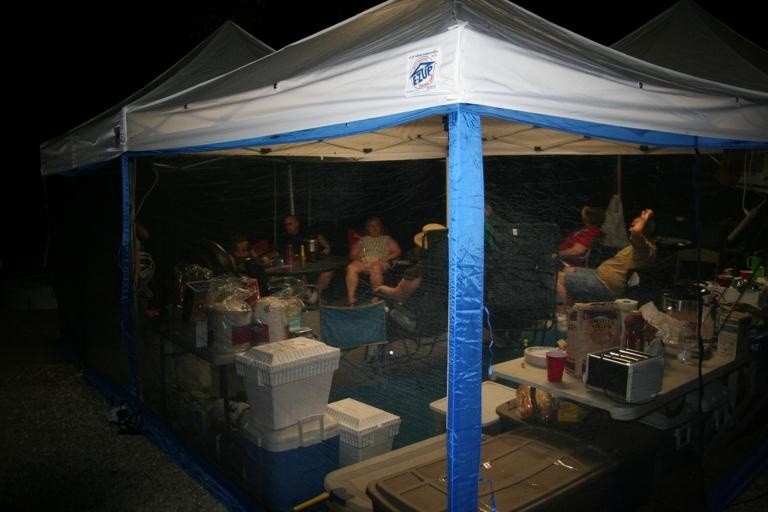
[
  {"xmin": 488, "ymin": 347, "xmax": 759, "ymax": 512},
  {"xmin": 150, "ymin": 323, "xmax": 315, "ymax": 473}
]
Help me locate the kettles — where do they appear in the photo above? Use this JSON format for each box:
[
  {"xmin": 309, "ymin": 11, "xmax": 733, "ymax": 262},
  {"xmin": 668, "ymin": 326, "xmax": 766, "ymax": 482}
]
[{"xmin": 661, "ymin": 284, "xmax": 707, "ymax": 333}]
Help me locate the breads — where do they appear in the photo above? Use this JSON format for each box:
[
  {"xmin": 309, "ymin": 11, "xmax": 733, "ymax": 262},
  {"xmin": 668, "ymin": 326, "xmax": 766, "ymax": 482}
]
[{"xmin": 517, "ymin": 383, "xmax": 554, "ymax": 422}]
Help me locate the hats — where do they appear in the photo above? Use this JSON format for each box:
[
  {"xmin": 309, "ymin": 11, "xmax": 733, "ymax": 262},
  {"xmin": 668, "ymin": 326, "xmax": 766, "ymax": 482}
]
[{"xmin": 414, "ymin": 223, "xmax": 446, "ymax": 249}]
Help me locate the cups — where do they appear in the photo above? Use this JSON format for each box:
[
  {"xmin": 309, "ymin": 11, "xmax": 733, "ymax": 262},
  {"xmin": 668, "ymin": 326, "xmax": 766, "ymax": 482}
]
[
  {"xmin": 546, "ymin": 350, "xmax": 568, "ymax": 382},
  {"xmin": 739, "ymin": 270, "xmax": 753, "ymax": 279},
  {"xmin": 717, "ymin": 275, "xmax": 732, "ymax": 287},
  {"xmin": 745, "ymin": 250, "xmax": 765, "ymax": 277}
]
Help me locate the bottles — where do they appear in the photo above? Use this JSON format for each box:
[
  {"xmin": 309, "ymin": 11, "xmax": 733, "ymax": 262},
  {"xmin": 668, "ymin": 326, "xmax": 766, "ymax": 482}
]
[
  {"xmin": 299, "ymin": 244, "xmax": 306, "ymax": 267},
  {"xmin": 309, "ymin": 240, "xmax": 316, "ymax": 263},
  {"xmin": 622, "ymin": 310, "xmax": 645, "ymax": 352}
]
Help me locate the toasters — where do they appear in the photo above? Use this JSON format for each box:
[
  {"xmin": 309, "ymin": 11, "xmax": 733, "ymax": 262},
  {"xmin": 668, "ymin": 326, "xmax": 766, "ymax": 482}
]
[{"xmin": 584, "ymin": 346, "xmax": 665, "ymax": 404}]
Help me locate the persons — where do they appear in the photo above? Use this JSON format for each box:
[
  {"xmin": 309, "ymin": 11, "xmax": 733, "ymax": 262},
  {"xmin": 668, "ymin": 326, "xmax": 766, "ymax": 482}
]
[
  {"xmin": 228, "ymin": 233, "xmax": 260, "ymax": 273},
  {"xmin": 343, "ymin": 217, "xmax": 400, "ymax": 307},
  {"xmin": 366, "ymin": 223, "xmax": 447, "ymax": 334},
  {"xmin": 134, "ymin": 219, "xmax": 149, "ymax": 244},
  {"xmin": 556, "ymin": 208, "xmax": 661, "ymax": 318},
  {"xmin": 281, "ymin": 214, "xmax": 336, "ymax": 305},
  {"xmin": 557, "ymin": 204, "xmax": 605, "ymax": 267}
]
